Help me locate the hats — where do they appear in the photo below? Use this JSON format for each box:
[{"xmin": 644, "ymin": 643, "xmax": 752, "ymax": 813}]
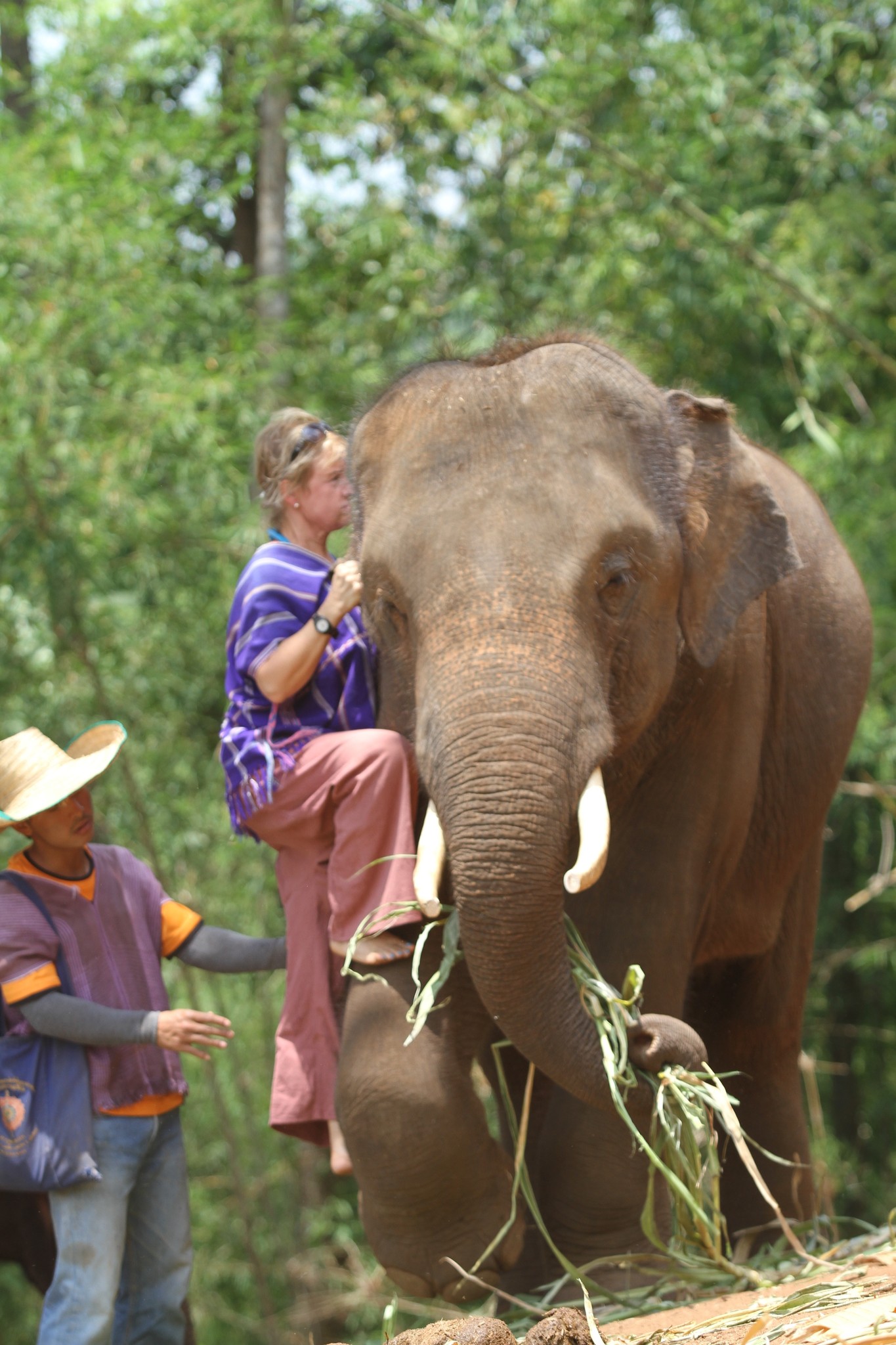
[{"xmin": 0, "ymin": 717, "xmax": 127, "ymax": 831}]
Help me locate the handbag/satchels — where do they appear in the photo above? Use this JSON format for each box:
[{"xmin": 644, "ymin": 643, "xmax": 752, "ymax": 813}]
[{"xmin": 0, "ymin": 868, "xmax": 106, "ymax": 1194}]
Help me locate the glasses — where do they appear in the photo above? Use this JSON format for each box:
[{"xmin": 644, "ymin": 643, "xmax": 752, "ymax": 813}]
[{"xmin": 287, "ymin": 421, "xmax": 336, "ymax": 463}]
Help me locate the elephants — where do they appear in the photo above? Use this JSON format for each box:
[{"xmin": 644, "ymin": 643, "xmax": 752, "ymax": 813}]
[{"xmin": 324, "ymin": 343, "xmax": 878, "ymax": 1303}]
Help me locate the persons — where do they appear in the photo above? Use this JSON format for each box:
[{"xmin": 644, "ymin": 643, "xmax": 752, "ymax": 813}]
[
  {"xmin": 1, "ymin": 719, "xmax": 284, "ymax": 1345},
  {"xmin": 224, "ymin": 405, "xmax": 420, "ymax": 1177}
]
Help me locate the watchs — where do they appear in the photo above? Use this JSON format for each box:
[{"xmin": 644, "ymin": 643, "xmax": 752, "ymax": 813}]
[{"xmin": 312, "ymin": 613, "xmax": 339, "ymax": 639}]
